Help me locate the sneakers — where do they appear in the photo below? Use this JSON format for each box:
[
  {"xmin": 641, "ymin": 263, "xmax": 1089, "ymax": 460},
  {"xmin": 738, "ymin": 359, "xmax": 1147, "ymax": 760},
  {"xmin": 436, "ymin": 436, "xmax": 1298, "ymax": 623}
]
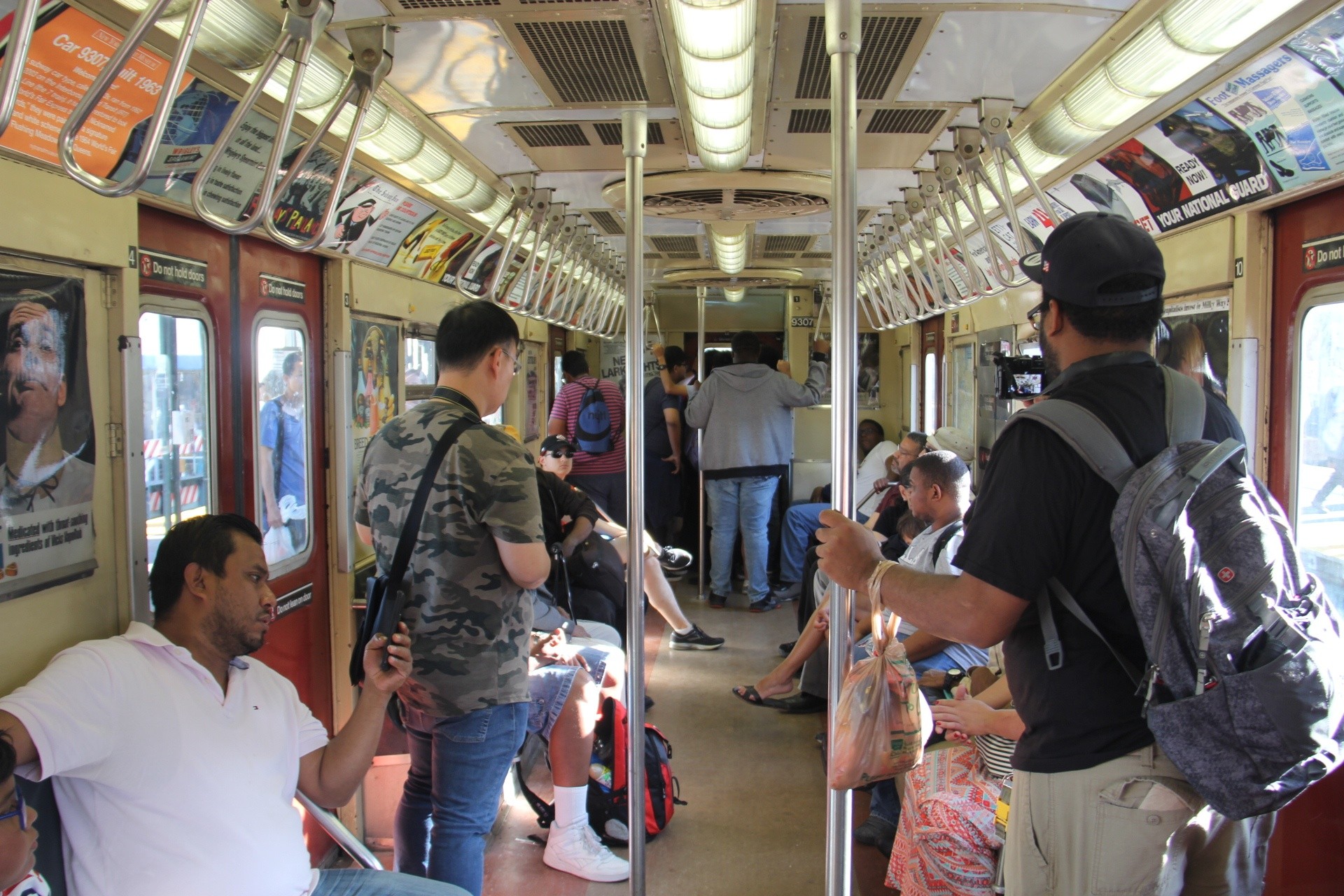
[
  {"xmin": 669, "ymin": 623, "xmax": 725, "ymax": 650},
  {"xmin": 706, "ymin": 591, "xmax": 727, "ymax": 608},
  {"xmin": 656, "ymin": 546, "xmax": 693, "ymax": 570},
  {"xmin": 543, "ymin": 812, "xmax": 629, "ymax": 882},
  {"xmin": 750, "ymin": 583, "xmax": 802, "ymax": 612}
]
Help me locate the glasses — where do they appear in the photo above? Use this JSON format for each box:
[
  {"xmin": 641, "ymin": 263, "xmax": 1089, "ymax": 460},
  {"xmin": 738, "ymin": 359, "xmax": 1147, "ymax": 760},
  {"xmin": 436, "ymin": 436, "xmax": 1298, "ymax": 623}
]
[
  {"xmin": 544, "ymin": 448, "xmax": 574, "ymax": 458},
  {"xmin": 858, "ymin": 427, "xmax": 878, "ymax": 437},
  {"xmin": 673, "ymin": 363, "xmax": 688, "ymax": 370},
  {"xmin": 0, "ymin": 785, "xmax": 27, "ymax": 831},
  {"xmin": 560, "ymin": 370, "xmax": 566, "ymax": 383},
  {"xmin": 490, "ymin": 348, "xmax": 522, "ymax": 375},
  {"xmin": 1027, "ymin": 302, "xmax": 1041, "ymax": 331},
  {"xmin": 897, "ymin": 443, "xmax": 935, "ymax": 456}
]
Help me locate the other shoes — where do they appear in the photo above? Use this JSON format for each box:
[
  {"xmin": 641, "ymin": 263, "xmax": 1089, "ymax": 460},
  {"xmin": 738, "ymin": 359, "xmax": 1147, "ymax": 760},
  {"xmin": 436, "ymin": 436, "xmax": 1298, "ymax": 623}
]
[
  {"xmin": 854, "ymin": 817, "xmax": 897, "ymax": 856},
  {"xmin": 665, "ymin": 569, "xmax": 789, "ymax": 594},
  {"xmin": 645, "ymin": 696, "xmax": 654, "ymax": 709},
  {"xmin": 779, "ymin": 640, "xmax": 827, "ymax": 775}
]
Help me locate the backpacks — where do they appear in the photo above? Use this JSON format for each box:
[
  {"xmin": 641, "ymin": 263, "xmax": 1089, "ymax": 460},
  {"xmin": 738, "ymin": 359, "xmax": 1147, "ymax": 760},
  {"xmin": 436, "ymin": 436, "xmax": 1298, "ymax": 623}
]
[
  {"xmin": 515, "ymin": 697, "xmax": 688, "ymax": 849},
  {"xmin": 566, "ymin": 378, "xmax": 624, "ymax": 454},
  {"xmin": 995, "ymin": 365, "xmax": 1344, "ymax": 821}
]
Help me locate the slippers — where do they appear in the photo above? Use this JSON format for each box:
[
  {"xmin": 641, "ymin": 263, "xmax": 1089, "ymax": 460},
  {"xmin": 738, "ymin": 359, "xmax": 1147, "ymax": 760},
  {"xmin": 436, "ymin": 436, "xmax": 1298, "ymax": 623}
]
[{"xmin": 733, "ymin": 685, "xmax": 792, "ymax": 710}]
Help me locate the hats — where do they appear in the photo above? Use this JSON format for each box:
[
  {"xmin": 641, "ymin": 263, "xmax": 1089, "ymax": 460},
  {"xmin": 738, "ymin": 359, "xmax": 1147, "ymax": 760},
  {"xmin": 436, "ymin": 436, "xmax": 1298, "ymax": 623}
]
[
  {"xmin": 541, "ymin": 434, "xmax": 577, "ymax": 456},
  {"xmin": 925, "ymin": 426, "xmax": 974, "ymax": 466},
  {"xmin": 1019, "ymin": 211, "xmax": 1166, "ymax": 307}
]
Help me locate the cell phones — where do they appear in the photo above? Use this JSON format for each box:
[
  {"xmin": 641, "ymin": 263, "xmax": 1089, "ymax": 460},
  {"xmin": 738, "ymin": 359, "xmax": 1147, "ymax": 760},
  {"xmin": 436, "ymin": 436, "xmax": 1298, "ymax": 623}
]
[{"xmin": 383, "ymin": 591, "xmax": 405, "ymax": 668}]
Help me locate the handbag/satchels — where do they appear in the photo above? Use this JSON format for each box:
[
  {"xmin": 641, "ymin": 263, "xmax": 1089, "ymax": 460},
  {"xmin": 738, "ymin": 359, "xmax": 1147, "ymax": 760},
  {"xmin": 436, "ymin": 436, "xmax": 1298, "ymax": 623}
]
[
  {"xmin": 830, "ymin": 559, "xmax": 934, "ymax": 791},
  {"xmin": 349, "ymin": 574, "xmax": 406, "ymax": 687}
]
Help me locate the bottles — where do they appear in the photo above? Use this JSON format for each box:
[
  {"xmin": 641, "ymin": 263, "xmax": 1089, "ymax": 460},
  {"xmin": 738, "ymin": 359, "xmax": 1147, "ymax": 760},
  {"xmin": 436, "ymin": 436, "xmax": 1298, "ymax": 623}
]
[
  {"xmin": 594, "ymin": 732, "xmax": 615, "ymax": 768},
  {"xmin": 1239, "ymin": 606, "xmax": 1314, "ymax": 670}
]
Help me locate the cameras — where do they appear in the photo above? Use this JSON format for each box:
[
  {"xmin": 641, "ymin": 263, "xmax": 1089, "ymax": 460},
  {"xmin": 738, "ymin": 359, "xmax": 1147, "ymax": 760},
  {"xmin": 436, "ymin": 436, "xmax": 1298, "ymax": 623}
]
[{"xmin": 993, "ymin": 355, "xmax": 1050, "ymax": 400}]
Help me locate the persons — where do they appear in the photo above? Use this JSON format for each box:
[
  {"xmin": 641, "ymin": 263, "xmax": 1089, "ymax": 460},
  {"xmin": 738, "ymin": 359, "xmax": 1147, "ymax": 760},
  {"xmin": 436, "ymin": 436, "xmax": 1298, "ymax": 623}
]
[
  {"xmin": 352, "ymin": 300, "xmax": 551, "ymax": 896},
  {"xmin": 1312, "ymin": 414, "xmax": 1344, "ymax": 514},
  {"xmin": 0, "ymin": 514, "xmax": 471, "ymax": 895},
  {"xmin": 813, "ymin": 213, "xmax": 1277, "ymax": 896},
  {"xmin": 522, "ymin": 330, "xmax": 1027, "ymax": 896},
  {"xmin": 0, "ymin": 728, "xmax": 55, "ymax": 895},
  {"xmin": 261, "ymin": 352, "xmax": 308, "ymax": 551},
  {"xmin": 1, "ymin": 288, "xmax": 94, "ymax": 515}
]
